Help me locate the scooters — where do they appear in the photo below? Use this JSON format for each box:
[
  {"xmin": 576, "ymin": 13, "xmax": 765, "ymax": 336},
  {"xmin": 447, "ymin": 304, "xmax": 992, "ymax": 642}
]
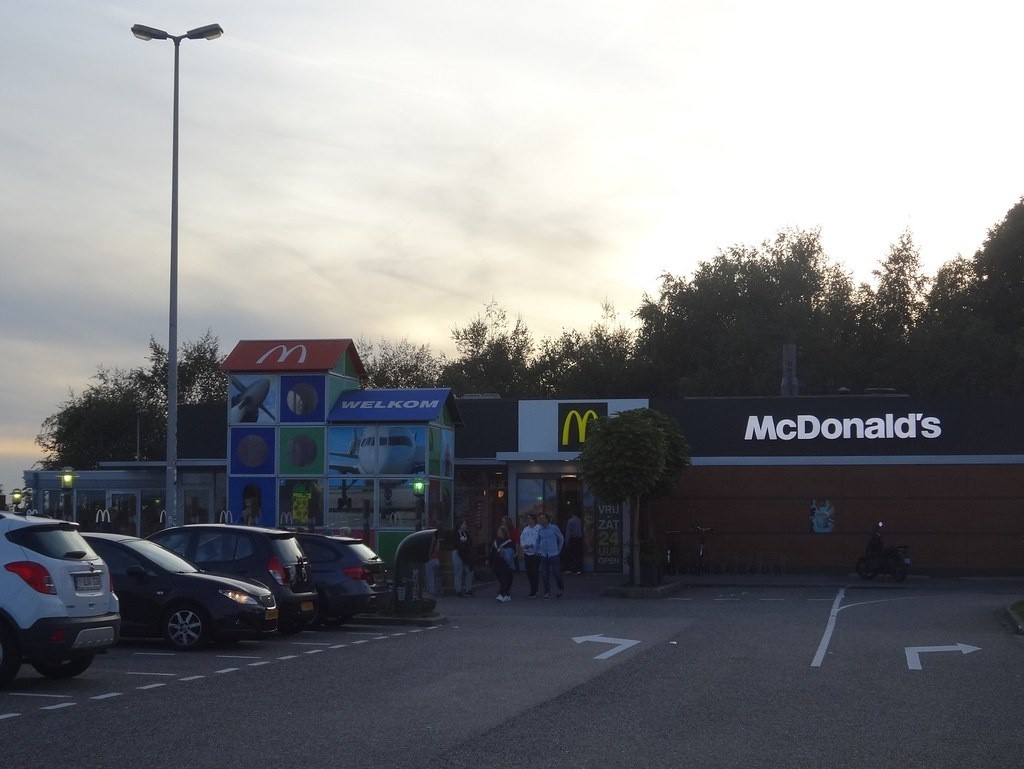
[{"xmin": 856, "ymin": 521, "xmax": 913, "ymax": 583}]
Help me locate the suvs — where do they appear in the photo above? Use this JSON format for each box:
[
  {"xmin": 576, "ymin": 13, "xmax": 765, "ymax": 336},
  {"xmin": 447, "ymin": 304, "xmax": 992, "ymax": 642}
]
[
  {"xmin": 0, "ymin": 510, "xmax": 121, "ymax": 686},
  {"xmin": 285, "ymin": 531, "xmax": 394, "ymax": 628},
  {"xmin": 129, "ymin": 524, "xmax": 322, "ymax": 634}
]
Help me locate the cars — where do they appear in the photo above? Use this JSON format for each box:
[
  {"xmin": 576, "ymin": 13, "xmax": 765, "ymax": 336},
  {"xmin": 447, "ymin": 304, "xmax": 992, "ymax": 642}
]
[{"xmin": 78, "ymin": 529, "xmax": 281, "ymax": 652}]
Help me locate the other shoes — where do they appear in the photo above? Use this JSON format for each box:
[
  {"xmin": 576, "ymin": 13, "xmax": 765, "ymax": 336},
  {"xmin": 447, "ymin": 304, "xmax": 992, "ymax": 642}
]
[
  {"xmin": 573, "ymin": 571, "xmax": 581, "ymax": 576},
  {"xmin": 528, "ymin": 591, "xmax": 539, "ymax": 598},
  {"xmin": 557, "ymin": 592, "xmax": 562, "ymax": 598},
  {"xmin": 496, "ymin": 594, "xmax": 503, "ymax": 602},
  {"xmin": 463, "ymin": 592, "xmax": 472, "ymax": 597},
  {"xmin": 562, "ymin": 571, "xmax": 570, "ymax": 575},
  {"xmin": 544, "ymin": 593, "xmax": 550, "ymax": 600},
  {"xmin": 503, "ymin": 595, "xmax": 511, "ymax": 601},
  {"xmin": 455, "ymin": 591, "xmax": 462, "ymax": 596}
]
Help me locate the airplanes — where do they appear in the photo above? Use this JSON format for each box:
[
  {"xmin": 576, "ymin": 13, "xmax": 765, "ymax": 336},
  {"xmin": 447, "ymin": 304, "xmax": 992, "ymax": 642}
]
[{"xmin": 329, "ymin": 424, "xmax": 427, "ymax": 516}]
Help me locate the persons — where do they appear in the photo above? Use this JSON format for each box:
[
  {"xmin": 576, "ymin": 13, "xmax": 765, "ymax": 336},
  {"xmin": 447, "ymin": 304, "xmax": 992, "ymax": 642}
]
[
  {"xmin": 450, "ymin": 518, "xmax": 476, "ymax": 599},
  {"xmin": 562, "ymin": 509, "xmax": 583, "ymax": 576},
  {"xmin": 94, "ymin": 506, "xmax": 136, "ymax": 536},
  {"xmin": 425, "ymin": 536, "xmax": 443, "ymax": 596},
  {"xmin": 484, "ymin": 513, "xmax": 565, "ymax": 604},
  {"xmin": 335, "ymin": 527, "xmax": 352, "ymax": 537}
]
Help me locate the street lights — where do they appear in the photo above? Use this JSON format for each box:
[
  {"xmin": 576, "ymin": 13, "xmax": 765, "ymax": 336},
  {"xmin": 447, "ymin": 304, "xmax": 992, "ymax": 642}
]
[{"xmin": 131, "ymin": 23, "xmax": 224, "ymax": 533}]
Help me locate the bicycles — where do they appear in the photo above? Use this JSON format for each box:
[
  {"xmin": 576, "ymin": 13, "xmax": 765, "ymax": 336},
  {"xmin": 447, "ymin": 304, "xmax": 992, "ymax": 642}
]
[
  {"xmin": 691, "ymin": 523, "xmax": 717, "ymax": 576},
  {"xmin": 657, "ymin": 529, "xmax": 681, "ymax": 577}
]
